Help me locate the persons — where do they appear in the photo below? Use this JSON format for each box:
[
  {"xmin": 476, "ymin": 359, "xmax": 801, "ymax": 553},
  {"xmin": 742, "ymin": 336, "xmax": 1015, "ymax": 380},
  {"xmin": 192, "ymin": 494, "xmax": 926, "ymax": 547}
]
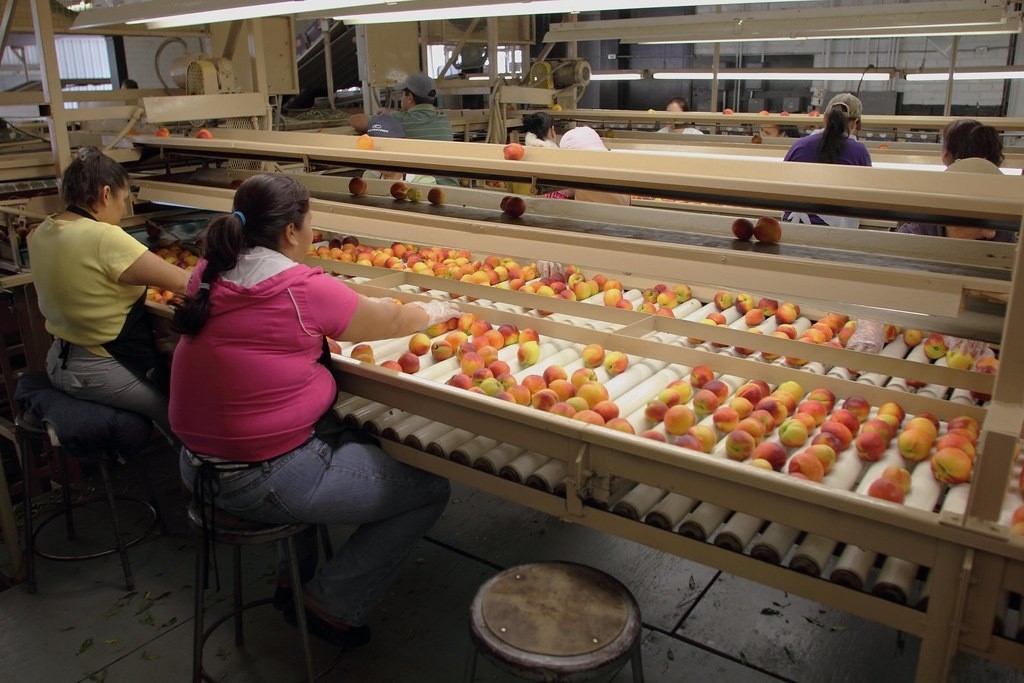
[
  {"xmin": 898, "ymin": 157, "xmax": 1017, "ymax": 242},
  {"xmin": 893, "ymin": 119, "xmax": 1005, "ymax": 232},
  {"xmin": 780, "ymin": 93, "xmax": 873, "ymax": 229},
  {"xmin": 653, "ymin": 97, "xmax": 704, "ymax": 134},
  {"xmin": 169, "ymin": 174, "xmax": 452, "ymax": 632},
  {"xmin": 525, "ymin": 113, "xmax": 558, "ymax": 148},
  {"xmin": 349, "ymin": 73, "xmax": 460, "ymax": 188},
  {"xmin": 26, "ymin": 150, "xmax": 193, "ymax": 425},
  {"xmin": 121, "ymin": 79, "xmax": 139, "ymax": 89}
]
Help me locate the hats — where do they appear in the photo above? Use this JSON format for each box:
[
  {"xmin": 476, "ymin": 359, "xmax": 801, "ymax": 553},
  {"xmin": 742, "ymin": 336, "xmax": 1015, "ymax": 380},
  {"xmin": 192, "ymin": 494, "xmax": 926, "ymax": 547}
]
[
  {"xmin": 945, "ymin": 157, "xmax": 1004, "ymax": 176},
  {"xmin": 367, "ymin": 114, "xmax": 406, "ymax": 138},
  {"xmin": 393, "ymin": 73, "xmax": 437, "ymax": 101},
  {"xmin": 824, "ymin": 92, "xmax": 862, "ymax": 131}
]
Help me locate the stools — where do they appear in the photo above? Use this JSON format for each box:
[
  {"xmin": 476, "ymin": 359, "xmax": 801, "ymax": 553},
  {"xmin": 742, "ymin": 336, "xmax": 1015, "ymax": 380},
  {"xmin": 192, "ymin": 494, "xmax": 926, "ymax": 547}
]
[
  {"xmin": 468, "ymin": 560, "xmax": 645, "ymax": 683},
  {"xmin": 15, "ymin": 408, "xmax": 169, "ymax": 593},
  {"xmin": 184, "ymin": 500, "xmax": 335, "ymax": 683}
]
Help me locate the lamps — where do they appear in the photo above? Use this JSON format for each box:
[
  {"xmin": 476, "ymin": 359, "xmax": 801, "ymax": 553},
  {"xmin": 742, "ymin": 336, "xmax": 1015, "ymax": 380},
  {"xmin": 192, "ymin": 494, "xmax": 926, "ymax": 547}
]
[
  {"xmin": 587, "ymin": 67, "xmax": 647, "ymax": 82},
  {"xmin": 902, "ymin": 64, "xmax": 1024, "ymax": 84},
  {"xmin": 651, "ymin": 67, "xmax": 898, "ymax": 84},
  {"xmin": 69, "ymin": 0, "xmax": 1022, "ymax": 44}
]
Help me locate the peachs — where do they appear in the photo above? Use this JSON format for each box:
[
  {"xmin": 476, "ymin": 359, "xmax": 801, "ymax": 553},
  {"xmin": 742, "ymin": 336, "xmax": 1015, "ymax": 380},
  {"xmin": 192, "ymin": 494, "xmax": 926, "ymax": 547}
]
[
  {"xmin": 504, "ymin": 143, "xmax": 526, "ymax": 160},
  {"xmin": 356, "ymin": 134, "xmax": 373, "ymax": 150},
  {"xmin": 310, "ymin": 176, "xmax": 999, "ymax": 504},
  {"xmin": 1010, "ymin": 440, "xmax": 1024, "ymax": 533},
  {"xmin": 723, "ymin": 108, "xmax": 820, "ymax": 144},
  {"xmin": 552, "ymin": 104, "xmax": 563, "ymax": 111},
  {"xmin": 596, "ymin": 129, "xmax": 616, "ymax": 139},
  {"xmin": 128, "ymin": 127, "xmax": 213, "ymax": 139},
  {"xmin": 143, "ymin": 226, "xmax": 199, "ymax": 304},
  {"xmin": 878, "ymin": 145, "xmax": 889, "ymax": 149}
]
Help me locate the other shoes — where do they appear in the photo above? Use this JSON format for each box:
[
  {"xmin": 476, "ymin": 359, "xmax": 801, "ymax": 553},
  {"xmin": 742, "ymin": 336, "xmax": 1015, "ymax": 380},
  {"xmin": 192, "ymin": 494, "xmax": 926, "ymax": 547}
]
[{"xmin": 272, "ymin": 593, "xmax": 372, "ymax": 649}]
